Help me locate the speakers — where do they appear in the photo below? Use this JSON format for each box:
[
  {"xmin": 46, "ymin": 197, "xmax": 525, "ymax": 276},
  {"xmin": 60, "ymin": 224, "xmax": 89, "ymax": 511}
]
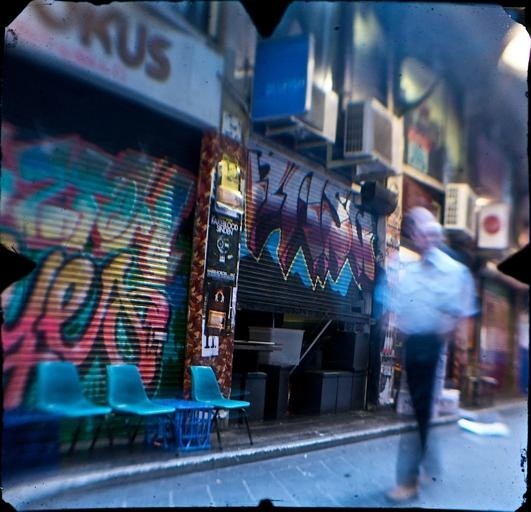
[{"xmin": 361, "ymin": 181, "xmax": 400, "ymax": 216}]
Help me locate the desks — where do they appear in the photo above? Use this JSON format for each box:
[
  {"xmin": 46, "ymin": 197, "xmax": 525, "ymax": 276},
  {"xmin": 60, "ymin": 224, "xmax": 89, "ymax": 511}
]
[{"xmin": 151, "ymin": 398, "xmax": 215, "ymax": 451}]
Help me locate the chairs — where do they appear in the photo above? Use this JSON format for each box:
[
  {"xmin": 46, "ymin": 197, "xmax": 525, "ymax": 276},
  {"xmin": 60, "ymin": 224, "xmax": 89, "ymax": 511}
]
[
  {"xmin": 187, "ymin": 365, "xmax": 254, "ymax": 450},
  {"xmin": 29, "ymin": 359, "xmax": 174, "ymax": 463}
]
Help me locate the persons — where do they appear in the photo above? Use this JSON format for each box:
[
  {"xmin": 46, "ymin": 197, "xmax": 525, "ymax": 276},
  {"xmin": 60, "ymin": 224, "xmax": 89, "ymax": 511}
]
[
  {"xmin": 1, "ymin": 243, "xmax": 37, "ymax": 511},
  {"xmin": 417, "ymin": 103, "xmax": 444, "ymax": 182}
]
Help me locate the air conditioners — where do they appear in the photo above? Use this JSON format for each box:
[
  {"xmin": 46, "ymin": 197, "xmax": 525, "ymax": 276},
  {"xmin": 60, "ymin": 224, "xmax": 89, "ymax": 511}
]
[
  {"xmin": 442, "ymin": 181, "xmax": 478, "ymax": 243},
  {"xmin": 292, "ymin": 84, "xmax": 403, "ymax": 179}
]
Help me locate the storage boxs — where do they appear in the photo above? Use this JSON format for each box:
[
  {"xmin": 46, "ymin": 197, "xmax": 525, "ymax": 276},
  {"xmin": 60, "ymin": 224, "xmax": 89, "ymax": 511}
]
[{"xmin": 250, "ymin": 327, "xmax": 305, "ymax": 368}]
